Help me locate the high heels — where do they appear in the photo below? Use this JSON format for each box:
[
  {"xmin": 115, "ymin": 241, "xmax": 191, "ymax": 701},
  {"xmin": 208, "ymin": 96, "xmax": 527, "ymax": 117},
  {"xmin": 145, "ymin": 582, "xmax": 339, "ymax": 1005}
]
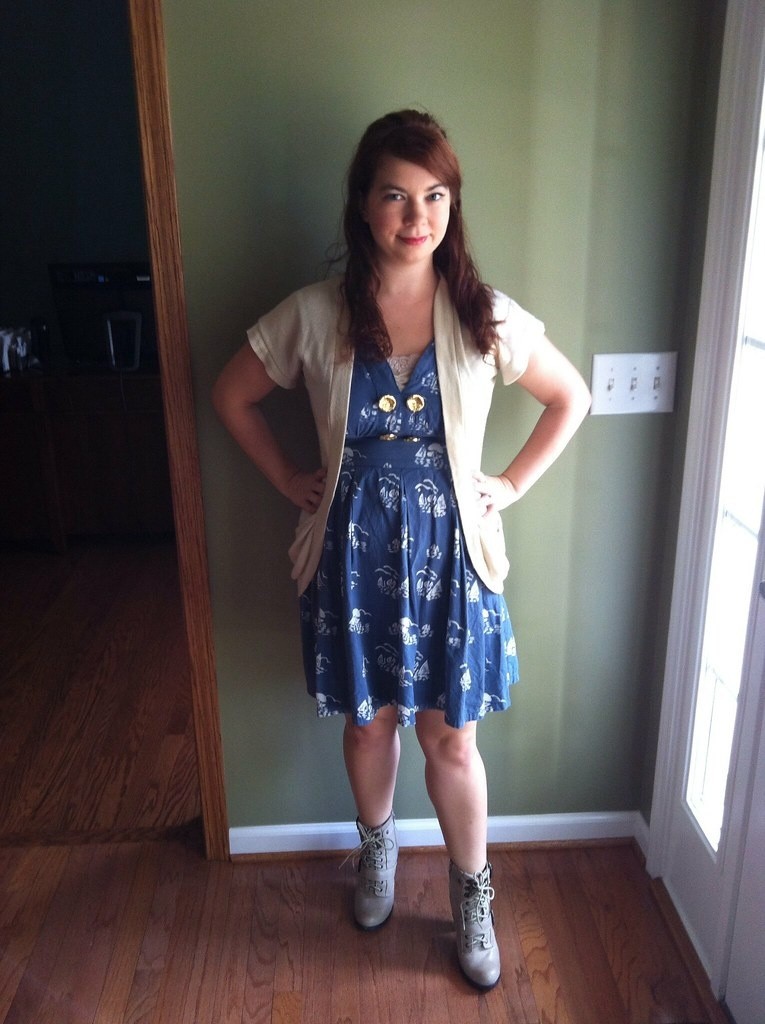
[
  {"xmin": 446, "ymin": 861, "xmax": 501, "ymax": 990},
  {"xmin": 351, "ymin": 814, "xmax": 396, "ymax": 931}
]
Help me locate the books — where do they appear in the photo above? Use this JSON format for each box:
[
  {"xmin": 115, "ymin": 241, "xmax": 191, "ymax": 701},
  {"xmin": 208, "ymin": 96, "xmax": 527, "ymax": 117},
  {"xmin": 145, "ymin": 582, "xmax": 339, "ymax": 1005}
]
[{"xmin": 0, "ymin": 324, "xmax": 38, "ymax": 380}]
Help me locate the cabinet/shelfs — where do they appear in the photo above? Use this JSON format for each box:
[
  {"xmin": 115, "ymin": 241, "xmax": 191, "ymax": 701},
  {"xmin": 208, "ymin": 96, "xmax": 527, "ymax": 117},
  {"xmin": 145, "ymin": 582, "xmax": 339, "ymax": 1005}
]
[{"xmin": 0, "ymin": 372, "xmax": 176, "ymax": 540}]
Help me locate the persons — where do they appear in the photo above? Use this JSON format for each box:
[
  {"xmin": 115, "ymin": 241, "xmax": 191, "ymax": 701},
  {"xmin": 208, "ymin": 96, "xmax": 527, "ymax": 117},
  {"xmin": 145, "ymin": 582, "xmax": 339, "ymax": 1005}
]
[{"xmin": 208, "ymin": 100, "xmax": 595, "ymax": 994}]
